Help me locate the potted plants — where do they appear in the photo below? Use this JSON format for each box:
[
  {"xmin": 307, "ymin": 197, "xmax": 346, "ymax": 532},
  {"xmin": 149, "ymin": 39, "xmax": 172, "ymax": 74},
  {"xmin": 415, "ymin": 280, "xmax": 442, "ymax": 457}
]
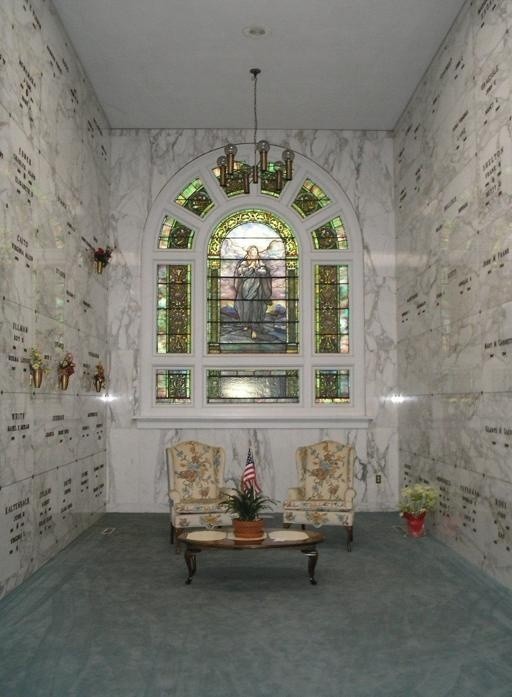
[{"xmin": 214, "ymin": 486, "xmax": 281, "ymax": 545}]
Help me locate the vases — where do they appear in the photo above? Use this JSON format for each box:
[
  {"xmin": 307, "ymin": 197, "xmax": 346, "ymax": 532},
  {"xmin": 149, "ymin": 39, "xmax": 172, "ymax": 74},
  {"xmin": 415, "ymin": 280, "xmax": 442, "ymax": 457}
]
[
  {"xmin": 60, "ymin": 374, "xmax": 69, "ymax": 391},
  {"xmin": 95, "ymin": 259, "xmax": 104, "ymax": 274},
  {"xmin": 33, "ymin": 369, "xmax": 43, "ymax": 388},
  {"xmin": 403, "ymin": 510, "xmax": 427, "ymax": 539},
  {"xmin": 96, "ymin": 379, "xmax": 102, "ymax": 392}
]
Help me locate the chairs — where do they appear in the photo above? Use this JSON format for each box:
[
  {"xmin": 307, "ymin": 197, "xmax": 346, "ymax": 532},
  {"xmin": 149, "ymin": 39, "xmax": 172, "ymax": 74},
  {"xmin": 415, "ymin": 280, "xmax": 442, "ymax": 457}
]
[
  {"xmin": 279, "ymin": 438, "xmax": 360, "ymax": 553},
  {"xmin": 164, "ymin": 441, "xmax": 233, "ymax": 545}
]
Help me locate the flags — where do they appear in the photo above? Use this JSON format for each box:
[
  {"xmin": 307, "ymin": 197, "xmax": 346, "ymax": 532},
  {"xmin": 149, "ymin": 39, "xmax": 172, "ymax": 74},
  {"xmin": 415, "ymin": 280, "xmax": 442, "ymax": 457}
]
[{"xmin": 240, "ymin": 450, "xmax": 263, "ymax": 497}]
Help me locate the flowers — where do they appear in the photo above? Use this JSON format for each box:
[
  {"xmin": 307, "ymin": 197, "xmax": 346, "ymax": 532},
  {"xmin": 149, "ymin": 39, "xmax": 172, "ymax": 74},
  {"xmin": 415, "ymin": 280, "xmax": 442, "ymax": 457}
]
[
  {"xmin": 58, "ymin": 352, "xmax": 77, "ymax": 376},
  {"xmin": 396, "ymin": 483, "xmax": 438, "ymax": 521},
  {"xmin": 28, "ymin": 346, "xmax": 51, "ymax": 374},
  {"xmin": 92, "ymin": 364, "xmax": 106, "ymax": 388},
  {"xmin": 94, "ymin": 244, "xmax": 114, "ymax": 267}
]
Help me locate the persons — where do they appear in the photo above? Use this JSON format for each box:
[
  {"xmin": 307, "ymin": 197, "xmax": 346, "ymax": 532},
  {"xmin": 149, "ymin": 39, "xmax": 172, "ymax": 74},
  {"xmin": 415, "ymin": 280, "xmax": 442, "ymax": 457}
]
[{"xmin": 233, "ymin": 245, "xmax": 274, "ymax": 339}]
